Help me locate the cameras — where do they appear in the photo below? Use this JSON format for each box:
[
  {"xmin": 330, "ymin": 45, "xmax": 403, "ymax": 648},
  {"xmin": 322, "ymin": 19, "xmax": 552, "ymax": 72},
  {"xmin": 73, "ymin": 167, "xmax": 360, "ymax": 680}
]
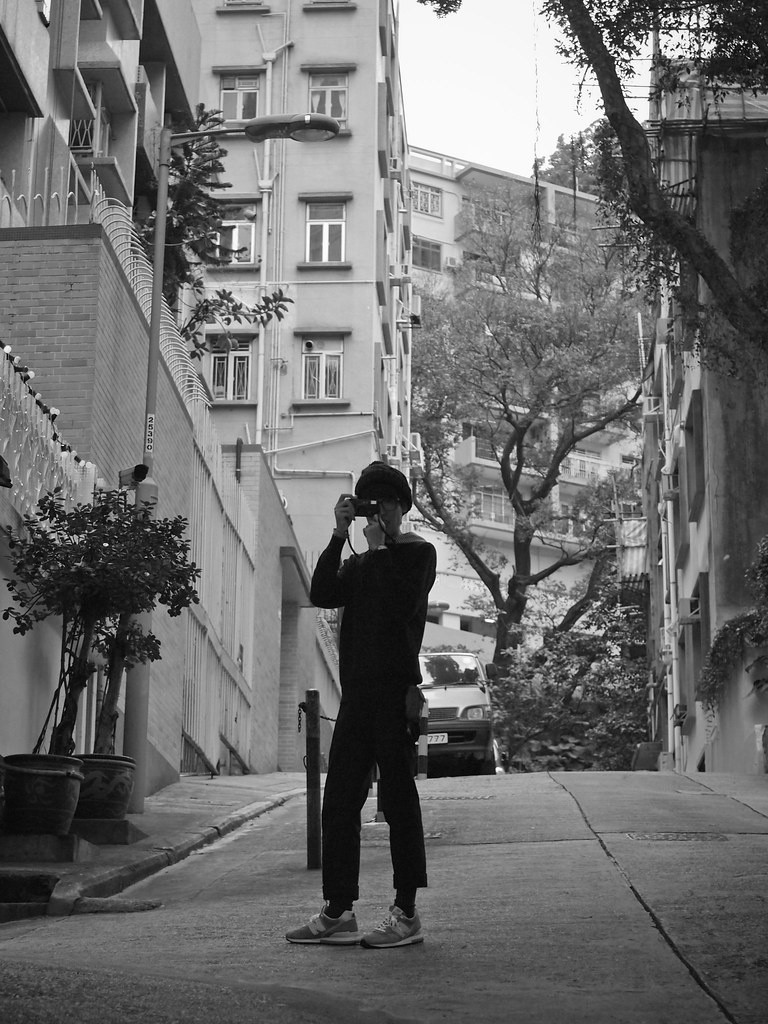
[{"xmin": 345, "ymin": 496, "xmax": 381, "ymax": 517}]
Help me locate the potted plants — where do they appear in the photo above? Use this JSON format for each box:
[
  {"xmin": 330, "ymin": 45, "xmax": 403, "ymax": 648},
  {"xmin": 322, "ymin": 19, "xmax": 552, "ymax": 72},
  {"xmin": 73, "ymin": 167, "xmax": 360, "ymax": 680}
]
[{"xmin": 0, "ymin": 488, "xmax": 203, "ymax": 834}]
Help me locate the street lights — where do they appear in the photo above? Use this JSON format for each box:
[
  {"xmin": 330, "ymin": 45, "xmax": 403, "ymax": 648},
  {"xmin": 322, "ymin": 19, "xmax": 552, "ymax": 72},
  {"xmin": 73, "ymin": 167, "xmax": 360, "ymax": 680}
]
[{"xmin": 120, "ymin": 111, "xmax": 342, "ymax": 813}]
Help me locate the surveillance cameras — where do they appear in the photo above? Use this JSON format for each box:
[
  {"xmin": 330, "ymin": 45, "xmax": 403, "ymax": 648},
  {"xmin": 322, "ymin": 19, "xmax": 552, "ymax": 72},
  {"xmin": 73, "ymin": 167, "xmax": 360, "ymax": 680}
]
[{"xmin": 120, "ymin": 463, "xmax": 149, "ymax": 487}]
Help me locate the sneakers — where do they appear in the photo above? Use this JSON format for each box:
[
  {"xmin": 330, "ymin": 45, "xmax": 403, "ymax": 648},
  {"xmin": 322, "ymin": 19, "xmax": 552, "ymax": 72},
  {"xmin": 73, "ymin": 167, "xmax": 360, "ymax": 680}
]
[
  {"xmin": 360, "ymin": 906, "xmax": 424, "ymax": 947},
  {"xmin": 285, "ymin": 906, "xmax": 361, "ymax": 943}
]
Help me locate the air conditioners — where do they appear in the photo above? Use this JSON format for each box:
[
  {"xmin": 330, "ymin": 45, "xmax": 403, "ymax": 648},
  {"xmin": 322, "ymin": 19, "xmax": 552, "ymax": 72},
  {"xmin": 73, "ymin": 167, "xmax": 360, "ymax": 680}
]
[
  {"xmin": 409, "ymin": 432, "xmax": 421, "ymax": 461},
  {"xmin": 391, "ymin": 158, "xmax": 401, "ymax": 178},
  {"xmin": 678, "ymin": 597, "xmax": 699, "ymax": 625},
  {"xmin": 412, "ymin": 448, "xmax": 425, "ymax": 476},
  {"xmin": 411, "ymin": 294, "xmax": 420, "ymax": 325},
  {"xmin": 402, "ymin": 264, "xmax": 411, "ymax": 282},
  {"xmin": 657, "ymin": 752, "xmax": 672, "ymax": 771}
]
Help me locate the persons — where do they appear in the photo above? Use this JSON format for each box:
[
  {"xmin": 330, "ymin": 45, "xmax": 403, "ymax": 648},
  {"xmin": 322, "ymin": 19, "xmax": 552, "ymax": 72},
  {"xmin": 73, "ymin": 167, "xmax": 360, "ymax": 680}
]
[{"xmin": 286, "ymin": 461, "xmax": 436, "ymax": 949}]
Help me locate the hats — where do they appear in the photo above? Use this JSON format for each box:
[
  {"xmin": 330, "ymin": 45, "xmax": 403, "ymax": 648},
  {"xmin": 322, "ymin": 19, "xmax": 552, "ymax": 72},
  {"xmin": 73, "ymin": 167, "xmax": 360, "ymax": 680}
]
[{"xmin": 355, "ymin": 461, "xmax": 412, "ymax": 516}]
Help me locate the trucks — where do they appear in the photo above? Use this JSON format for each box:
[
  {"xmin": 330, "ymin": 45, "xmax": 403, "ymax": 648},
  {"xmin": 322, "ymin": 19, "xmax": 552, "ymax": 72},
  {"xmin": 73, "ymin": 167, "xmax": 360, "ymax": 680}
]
[{"xmin": 420, "ymin": 652, "xmax": 497, "ymax": 776}]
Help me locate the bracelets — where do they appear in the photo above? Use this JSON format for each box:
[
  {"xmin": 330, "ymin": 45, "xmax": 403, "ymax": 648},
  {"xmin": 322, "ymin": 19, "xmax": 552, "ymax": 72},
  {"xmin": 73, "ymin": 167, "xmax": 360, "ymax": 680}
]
[
  {"xmin": 371, "ymin": 544, "xmax": 388, "ymax": 552},
  {"xmin": 334, "ymin": 528, "xmax": 347, "ymax": 537}
]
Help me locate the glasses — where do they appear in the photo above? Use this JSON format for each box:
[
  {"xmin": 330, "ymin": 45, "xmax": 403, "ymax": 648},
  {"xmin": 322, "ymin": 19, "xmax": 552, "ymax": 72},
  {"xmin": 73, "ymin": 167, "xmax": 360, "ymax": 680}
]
[{"xmin": 382, "ymin": 497, "xmax": 402, "ymax": 510}]
